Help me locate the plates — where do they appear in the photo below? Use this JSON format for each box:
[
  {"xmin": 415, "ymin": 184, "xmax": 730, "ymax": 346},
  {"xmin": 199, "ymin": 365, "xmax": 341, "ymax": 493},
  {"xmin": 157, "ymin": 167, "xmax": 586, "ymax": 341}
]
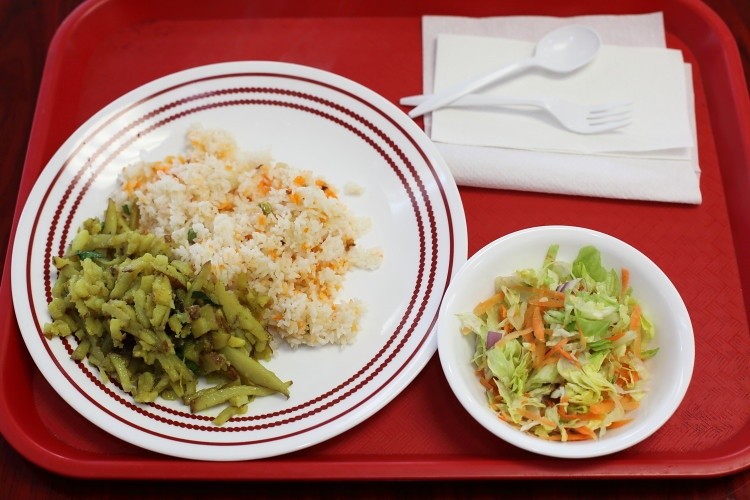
[
  {"xmin": 437, "ymin": 224, "xmax": 695, "ymax": 460},
  {"xmin": 10, "ymin": 61, "xmax": 468, "ymax": 463}
]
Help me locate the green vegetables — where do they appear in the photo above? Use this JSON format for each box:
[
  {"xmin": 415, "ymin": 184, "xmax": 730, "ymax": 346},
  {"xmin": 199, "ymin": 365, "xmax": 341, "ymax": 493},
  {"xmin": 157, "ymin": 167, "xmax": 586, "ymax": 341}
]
[{"xmin": 458, "ymin": 243, "xmax": 659, "ymax": 442}]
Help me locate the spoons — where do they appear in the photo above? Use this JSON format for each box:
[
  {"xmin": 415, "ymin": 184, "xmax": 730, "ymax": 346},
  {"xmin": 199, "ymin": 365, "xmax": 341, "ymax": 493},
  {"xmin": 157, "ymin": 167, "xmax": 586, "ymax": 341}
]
[{"xmin": 407, "ymin": 23, "xmax": 603, "ymax": 119}]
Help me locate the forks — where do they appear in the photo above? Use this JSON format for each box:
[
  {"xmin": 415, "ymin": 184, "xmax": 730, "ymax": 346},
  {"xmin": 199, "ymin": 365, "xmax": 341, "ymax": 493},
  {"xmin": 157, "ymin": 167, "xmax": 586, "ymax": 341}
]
[{"xmin": 399, "ymin": 93, "xmax": 634, "ymax": 133}]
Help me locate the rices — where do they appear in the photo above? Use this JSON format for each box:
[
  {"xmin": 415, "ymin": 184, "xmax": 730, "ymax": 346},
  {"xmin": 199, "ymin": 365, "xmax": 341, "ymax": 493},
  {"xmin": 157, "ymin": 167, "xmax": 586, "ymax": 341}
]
[{"xmin": 119, "ymin": 128, "xmax": 381, "ymax": 349}]
